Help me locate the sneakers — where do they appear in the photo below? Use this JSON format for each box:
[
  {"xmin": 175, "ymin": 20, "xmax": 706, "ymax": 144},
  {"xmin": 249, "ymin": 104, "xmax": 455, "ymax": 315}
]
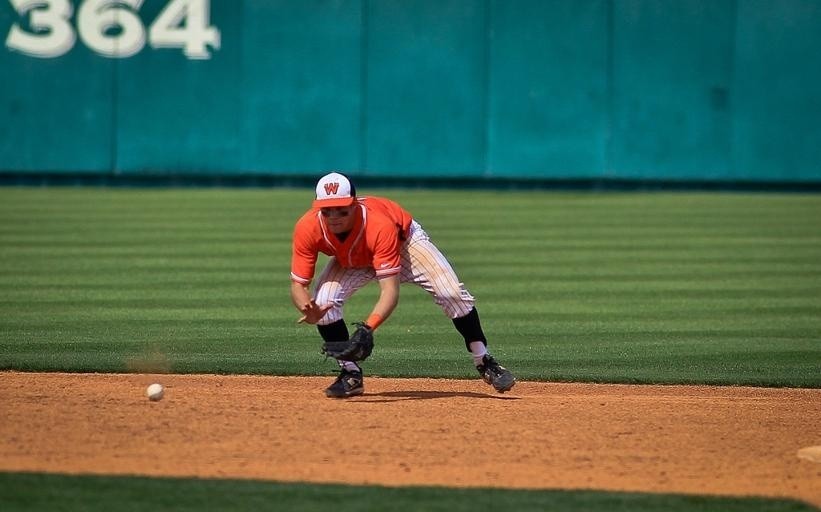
[
  {"xmin": 325, "ymin": 367, "xmax": 364, "ymax": 397},
  {"xmin": 476, "ymin": 354, "xmax": 515, "ymax": 393}
]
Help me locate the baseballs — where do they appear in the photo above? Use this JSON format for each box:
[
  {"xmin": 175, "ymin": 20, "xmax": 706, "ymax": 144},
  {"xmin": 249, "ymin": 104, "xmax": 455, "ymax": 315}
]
[{"xmin": 146, "ymin": 384, "xmax": 164, "ymax": 400}]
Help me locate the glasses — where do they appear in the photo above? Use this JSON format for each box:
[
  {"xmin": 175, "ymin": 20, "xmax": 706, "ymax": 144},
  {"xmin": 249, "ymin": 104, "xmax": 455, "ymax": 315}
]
[{"xmin": 321, "ymin": 211, "xmax": 350, "ymax": 217}]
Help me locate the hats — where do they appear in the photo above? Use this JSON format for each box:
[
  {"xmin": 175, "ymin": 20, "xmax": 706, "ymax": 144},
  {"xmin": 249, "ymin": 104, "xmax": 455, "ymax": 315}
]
[{"xmin": 312, "ymin": 172, "xmax": 355, "ymax": 208}]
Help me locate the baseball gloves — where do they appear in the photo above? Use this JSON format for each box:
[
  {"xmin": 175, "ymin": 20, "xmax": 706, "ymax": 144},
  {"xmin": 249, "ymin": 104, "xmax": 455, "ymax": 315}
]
[{"xmin": 321, "ymin": 321, "xmax": 375, "ymax": 363}]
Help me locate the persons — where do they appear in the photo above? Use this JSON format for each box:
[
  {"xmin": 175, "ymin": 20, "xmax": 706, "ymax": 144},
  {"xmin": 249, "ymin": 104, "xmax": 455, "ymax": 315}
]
[{"xmin": 287, "ymin": 170, "xmax": 519, "ymax": 399}]
